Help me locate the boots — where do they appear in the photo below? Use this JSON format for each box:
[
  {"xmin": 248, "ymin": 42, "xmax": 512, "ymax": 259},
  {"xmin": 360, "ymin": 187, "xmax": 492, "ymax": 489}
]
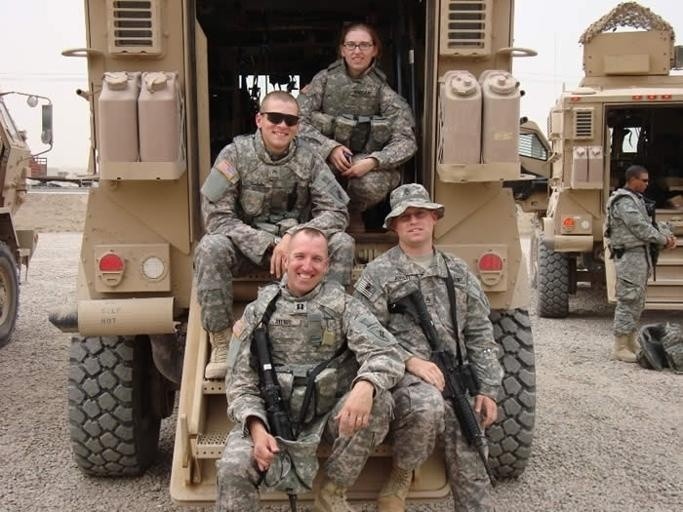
[
  {"xmin": 347, "ymin": 209, "xmax": 366, "ymax": 233},
  {"xmin": 205, "ymin": 326, "xmax": 233, "ymax": 379},
  {"xmin": 315, "ymin": 475, "xmax": 356, "ymax": 512},
  {"xmin": 378, "ymin": 462, "xmax": 413, "ymax": 512},
  {"xmin": 614, "ymin": 331, "xmax": 641, "ymax": 363}
]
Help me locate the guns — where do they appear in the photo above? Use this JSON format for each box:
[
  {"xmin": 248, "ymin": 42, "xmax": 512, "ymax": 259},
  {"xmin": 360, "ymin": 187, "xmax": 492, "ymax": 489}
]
[
  {"xmin": 649, "ymin": 205, "xmax": 660, "ymax": 281},
  {"xmin": 250, "ymin": 330, "xmax": 297, "ymax": 512},
  {"xmin": 388, "ymin": 289, "xmax": 496, "ymax": 488}
]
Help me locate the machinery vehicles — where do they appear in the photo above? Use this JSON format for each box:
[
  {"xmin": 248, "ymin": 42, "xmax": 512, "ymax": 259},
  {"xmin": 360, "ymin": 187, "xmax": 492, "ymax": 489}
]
[
  {"xmin": 49, "ymin": 0, "xmax": 536, "ymax": 509},
  {"xmin": 519, "ymin": 1, "xmax": 683, "ymax": 317},
  {"xmin": 0, "ymin": 91, "xmax": 57, "ymax": 344}
]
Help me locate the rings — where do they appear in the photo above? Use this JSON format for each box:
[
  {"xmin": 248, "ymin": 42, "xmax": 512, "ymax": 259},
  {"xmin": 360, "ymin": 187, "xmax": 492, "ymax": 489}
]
[{"xmin": 356, "ymin": 417, "xmax": 362, "ymax": 421}]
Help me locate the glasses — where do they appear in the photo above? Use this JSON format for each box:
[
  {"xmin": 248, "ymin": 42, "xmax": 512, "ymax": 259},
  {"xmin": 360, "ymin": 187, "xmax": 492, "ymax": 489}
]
[
  {"xmin": 641, "ymin": 179, "xmax": 649, "ymax": 182},
  {"xmin": 260, "ymin": 112, "xmax": 299, "ymax": 126},
  {"xmin": 343, "ymin": 43, "xmax": 374, "ymax": 50}
]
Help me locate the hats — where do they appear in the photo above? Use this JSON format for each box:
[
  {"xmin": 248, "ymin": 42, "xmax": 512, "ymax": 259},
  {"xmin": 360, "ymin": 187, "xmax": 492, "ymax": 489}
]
[
  {"xmin": 264, "ymin": 434, "xmax": 321, "ymax": 494},
  {"xmin": 382, "ymin": 183, "xmax": 444, "ymax": 230}
]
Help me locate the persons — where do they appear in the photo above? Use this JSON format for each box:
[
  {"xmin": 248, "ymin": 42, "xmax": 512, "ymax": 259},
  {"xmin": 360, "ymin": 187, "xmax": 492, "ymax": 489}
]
[
  {"xmin": 351, "ymin": 182, "xmax": 505, "ymax": 512},
  {"xmin": 193, "ymin": 90, "xmax": 356, "ymax": 379},
  {"xmin": 295, "ymin": 21, "xmax": 418, "ymax": 211},
  {"xmin": 603, "ymin": 166, "xmax": 676, "ymax": 362},
  {"xmin": 216, "ymin": 226, "xmax": 406, "ymax": 512}
]
